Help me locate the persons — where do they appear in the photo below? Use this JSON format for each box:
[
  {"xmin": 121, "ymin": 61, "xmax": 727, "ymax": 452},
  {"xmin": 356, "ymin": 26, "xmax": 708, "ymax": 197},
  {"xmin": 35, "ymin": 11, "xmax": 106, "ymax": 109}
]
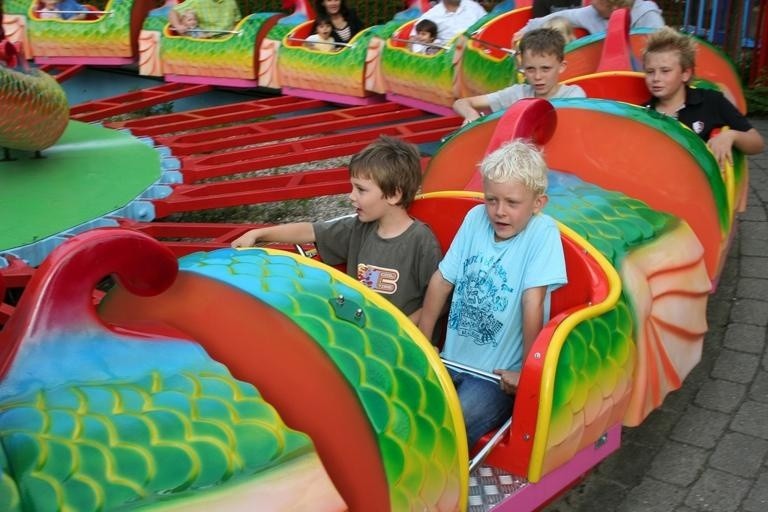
[
  {"xmin": 57, "ymin": 0, "xmax": 90, "ymax": 20},
  {"xmin": 39, "ymin": 0, "xmax": 61, "ymax": 19},
  {"xmin": 530, "ymin": 0, "xmax": 585, "ymax": 18},
  {"xmin": 311, "ymin": 0, "xmax": 361, "ymax": 47},
  {"xmin": 636, "ymin": 27, "xmax": 764, "ymax": 176},
  {"xmin": 167, "ymin": 0, "xmax": 243, "ymax": 38},
  {"xmin": 448, "ymin": 24, "xmax": 590, "ymax": 127},
  {"xmin": 401, "ymin": 0, "xmax": 489, "ymax": 49},
  {"xmin": 179, "ymin": 9, "xmax": 203, "ymax": 40},
  {"xmin": 415, "ymin": 139, "xmax": 567, "ymax": 455},
  {"xmin": 228, "ymin": 134, "xmax": 452, "ymax": 348},
  {"xmin": 407, "ymin": 18, "xmax": 444, "ymax": 57},
  {"xmin": 509, "ymin": 0, "xmax": 669, "ymax": 46},
  {"xmin": 302, "ymin": 16, "xmax": 342, "ymax": 51}
]
